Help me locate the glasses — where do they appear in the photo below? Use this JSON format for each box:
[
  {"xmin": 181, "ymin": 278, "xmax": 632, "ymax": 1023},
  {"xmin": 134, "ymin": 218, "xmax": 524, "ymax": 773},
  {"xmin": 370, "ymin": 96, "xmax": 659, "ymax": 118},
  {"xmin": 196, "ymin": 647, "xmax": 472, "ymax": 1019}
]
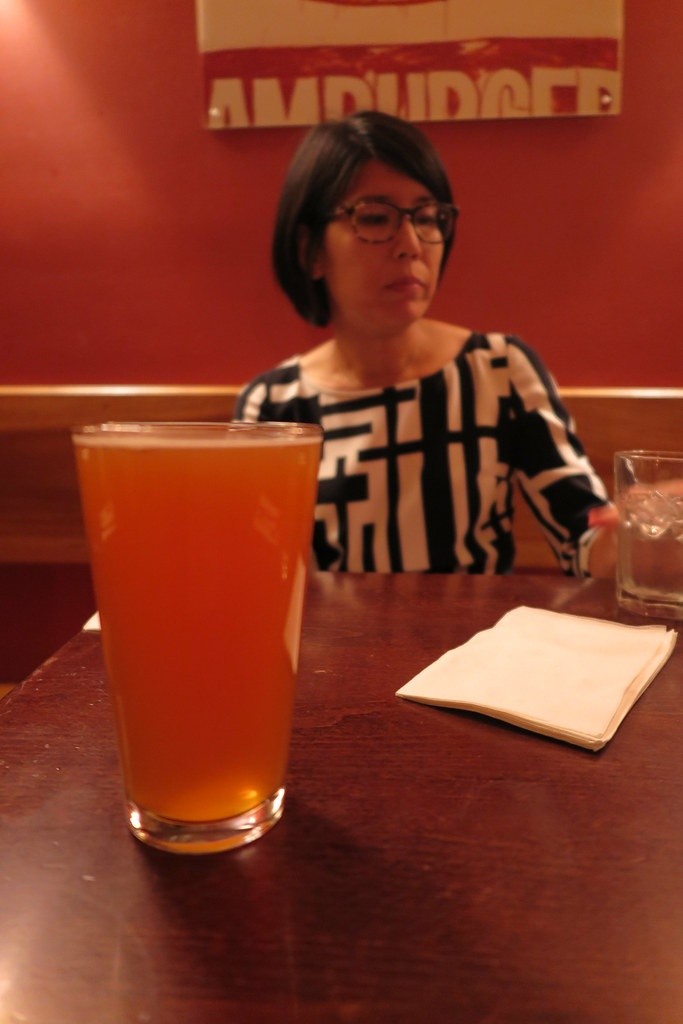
[{"xmin": 325, "ymin": 198, "xmax": 458, "ymax": 245}]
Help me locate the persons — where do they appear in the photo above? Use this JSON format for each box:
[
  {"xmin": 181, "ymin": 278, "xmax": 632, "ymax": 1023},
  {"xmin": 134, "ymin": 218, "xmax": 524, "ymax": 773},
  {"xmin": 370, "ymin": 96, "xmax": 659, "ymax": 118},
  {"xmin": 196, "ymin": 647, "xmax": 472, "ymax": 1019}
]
[{"xmin": 230, "ymin": 109, "xmax": 683, "ymax": 583}]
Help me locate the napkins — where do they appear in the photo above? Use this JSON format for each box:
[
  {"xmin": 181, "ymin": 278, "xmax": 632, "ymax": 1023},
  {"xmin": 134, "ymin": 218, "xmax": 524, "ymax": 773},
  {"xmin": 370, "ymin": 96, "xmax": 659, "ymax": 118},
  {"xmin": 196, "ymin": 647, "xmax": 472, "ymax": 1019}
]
[{"xmin": 394, "ymin": 606, "xmax": 677, "ymax": 752}]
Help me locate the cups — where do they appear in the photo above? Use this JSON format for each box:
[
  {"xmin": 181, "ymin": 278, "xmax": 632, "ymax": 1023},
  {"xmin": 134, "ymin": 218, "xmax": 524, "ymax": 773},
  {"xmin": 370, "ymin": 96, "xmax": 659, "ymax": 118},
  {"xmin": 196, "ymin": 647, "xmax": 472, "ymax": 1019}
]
[
  {"xmin": 612, "ymin": 448, "xmax": 683, "ymax": 621},
  {"xmin": 69, "ymin": 419, "xmax": 324, "ymax": 858}
]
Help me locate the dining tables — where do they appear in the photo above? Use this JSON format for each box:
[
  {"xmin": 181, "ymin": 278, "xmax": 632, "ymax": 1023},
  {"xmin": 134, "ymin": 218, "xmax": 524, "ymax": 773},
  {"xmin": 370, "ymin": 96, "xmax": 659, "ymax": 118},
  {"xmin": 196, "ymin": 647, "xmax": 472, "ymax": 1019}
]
[{"xmin": 0, "ymin": 571, "xmax": 683, "ymax": 1024}]
